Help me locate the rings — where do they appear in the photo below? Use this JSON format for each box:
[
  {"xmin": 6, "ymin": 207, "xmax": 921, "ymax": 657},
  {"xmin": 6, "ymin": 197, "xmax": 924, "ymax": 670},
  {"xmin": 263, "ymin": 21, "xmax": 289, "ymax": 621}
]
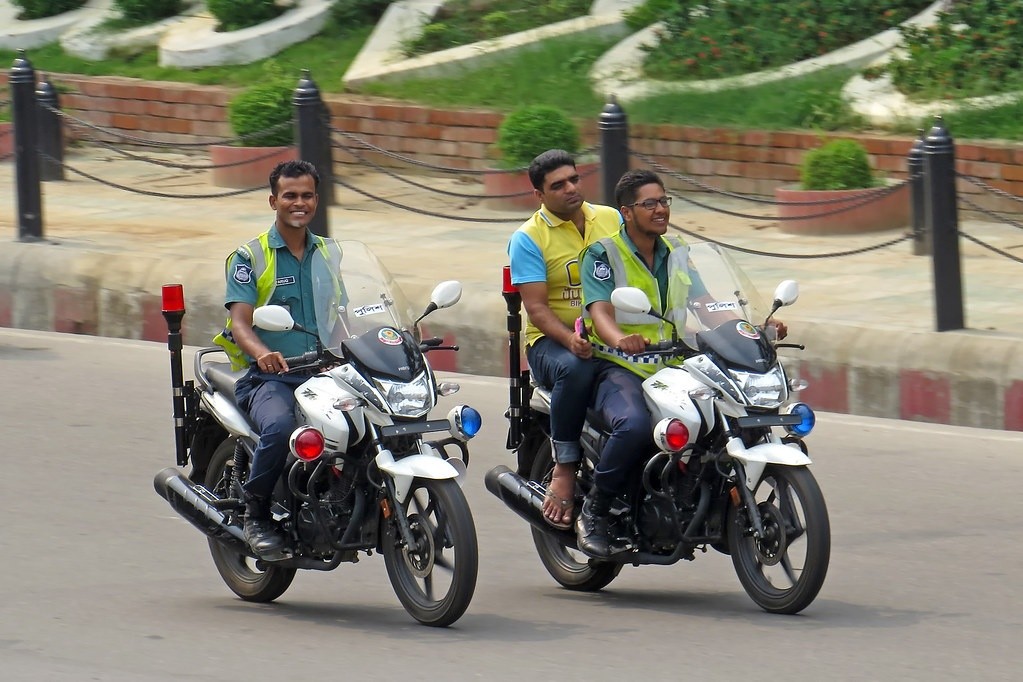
[{"xmin": 267, "ymin": 365, "xmax": 273, "ymax": 368}]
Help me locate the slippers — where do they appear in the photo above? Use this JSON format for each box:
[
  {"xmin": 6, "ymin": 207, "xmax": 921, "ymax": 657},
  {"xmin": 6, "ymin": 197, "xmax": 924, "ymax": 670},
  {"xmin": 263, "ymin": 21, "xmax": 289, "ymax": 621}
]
[{"xmin": 542, "ymin": 482, "xmax": 572, "ymax": 530}]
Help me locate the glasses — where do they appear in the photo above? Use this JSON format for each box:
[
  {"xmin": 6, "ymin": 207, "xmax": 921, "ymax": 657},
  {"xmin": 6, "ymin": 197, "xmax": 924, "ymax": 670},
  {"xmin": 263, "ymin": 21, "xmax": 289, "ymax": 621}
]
[{"xmin": 627, "ymin": 197, "xmax": 672, "ymax": 210}]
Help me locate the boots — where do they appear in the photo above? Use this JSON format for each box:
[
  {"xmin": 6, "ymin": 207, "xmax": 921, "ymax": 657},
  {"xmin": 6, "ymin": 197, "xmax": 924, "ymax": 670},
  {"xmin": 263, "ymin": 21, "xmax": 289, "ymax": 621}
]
[
  {"xmin": 574, "ymin": 484, "xmax": 611, "ymax": 560},
  {"xmin": 243, "ymin": 489, "xmax": 285, "ymax": 556}
]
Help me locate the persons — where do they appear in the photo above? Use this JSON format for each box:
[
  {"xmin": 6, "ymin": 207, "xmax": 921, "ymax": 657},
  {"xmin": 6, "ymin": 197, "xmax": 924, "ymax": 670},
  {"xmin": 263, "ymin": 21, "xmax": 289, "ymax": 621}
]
[
  {"xmin": 575, "ymin": 170, "xmax": 787, "ymax": 560},
  {"xmin": 507, "ymin": 149, "xmax": 624, "ymax": 529},
  {"xmin": 211, "ymin": 160, "xmax": 351, "ymax": 556}
]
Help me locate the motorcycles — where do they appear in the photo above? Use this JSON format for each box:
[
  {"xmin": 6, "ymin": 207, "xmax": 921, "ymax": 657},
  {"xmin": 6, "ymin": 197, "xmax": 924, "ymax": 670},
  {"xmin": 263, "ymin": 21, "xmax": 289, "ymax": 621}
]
[
  {"xmin": 482, "ymin": 240, "xmax": 830, "ymax": 615},
  {"xmin": 151, "ymin": 240, "xmax": 484, "ymax": 627}
]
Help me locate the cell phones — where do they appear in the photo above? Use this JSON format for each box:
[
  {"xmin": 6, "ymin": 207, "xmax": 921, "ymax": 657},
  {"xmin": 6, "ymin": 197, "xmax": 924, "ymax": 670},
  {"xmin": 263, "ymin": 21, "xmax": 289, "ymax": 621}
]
[{"xmin": 579, "ymin": 317, "xmax": 590, "ymax": 343}]
